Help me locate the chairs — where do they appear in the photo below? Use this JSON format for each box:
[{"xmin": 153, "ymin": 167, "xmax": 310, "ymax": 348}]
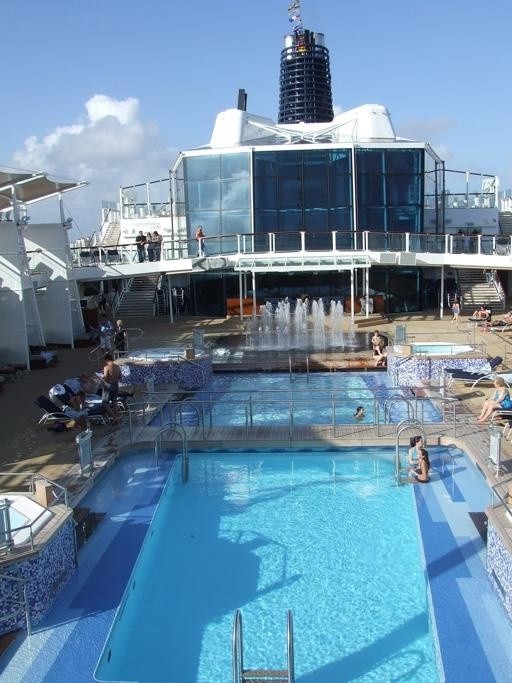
[
  {"xmin": 443, "ymin": 308, "xmax": 512, "ymax": 446},
  {"xmin": 80, "ymin": 249, "xmax": 126, "ymax": 266},
  {"xmin": 37, "ymin": 369, "xmax": 136, "ymax": 439},
  {"xmin": 0, "ymin": 363, "xmax": 28, "ymax": 387}
]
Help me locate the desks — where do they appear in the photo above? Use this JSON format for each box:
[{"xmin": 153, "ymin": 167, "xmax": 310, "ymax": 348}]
[
  {"xmin": 227, "ymin": 298, "xmax": 265, "ymax": 315},
  {"xmin": 344, "ymin": 295, "xmax": 384, "ymax": 313}
]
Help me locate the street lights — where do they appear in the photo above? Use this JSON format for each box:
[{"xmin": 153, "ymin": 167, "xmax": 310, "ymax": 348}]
[{"xmin": 144, "ymin": 176, "xmax": 151, "ymax": 215}]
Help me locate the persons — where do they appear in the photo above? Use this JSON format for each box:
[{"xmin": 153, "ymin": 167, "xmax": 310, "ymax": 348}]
[
  {"xmin": 195, "ymin": 224, "xmax": 208, "ymax": 256},
  {"xmin": 69, "ymin": 389, "xmax": 118, "ymax": 423},
  {"xmin": 152, "ymin": 230, "xmax": 164, "ymax": 261},
  {"xmin": 145, "ymin": 231, "xmax": 155, "ymax": 261},
  {"xmin": 470, "ymin": 303, "xmax": 491, "ymax": 320},
  {"xmin": 136, "ymin": 230, "xmax": 147, "ymax": 262},
  {"xmin": 99, "ymin": 353, "xmax": 123, "ymax": 423},
  {"xmin": 450, "ymin": 299, "xmax": 460, "ymax": 323},
  {"xmin": 394, "ymin": 447, "xmax": 431, "ymax": 484},
  {"xmin": 96, "ymin": 312, "xmax": 115, "ymax": 355},
  {"xmin": 112, "ymin": 318, "xmax": 127, "ymax": 357},
  {"xmin": 470, "ymin": 228, "xmax": 483, "ymax": 253},
  {"xmin": 398, "ymin": 434, "xmax": 424, "ymax": 473},
  {"xmin": 79, "ymin": 373, "xmax": 136, "ymax": 396},
  {"xmin": 457, "ymin": 228, "xmax": 473, "ymax": 253},
  {"xmin": 353, "ymin": 406, "xmax": 365, "ymax": 418},
  {"xmin": 370, "ymin": 329, "xmax": 383, "ymax": 354},
  {"xmin": 473, "ymin": 376, "xmax": 512, "ymax": 422},
  {"xmin": 484, "ymin": 310, "xmax": 512, "ymax": 326}
]
